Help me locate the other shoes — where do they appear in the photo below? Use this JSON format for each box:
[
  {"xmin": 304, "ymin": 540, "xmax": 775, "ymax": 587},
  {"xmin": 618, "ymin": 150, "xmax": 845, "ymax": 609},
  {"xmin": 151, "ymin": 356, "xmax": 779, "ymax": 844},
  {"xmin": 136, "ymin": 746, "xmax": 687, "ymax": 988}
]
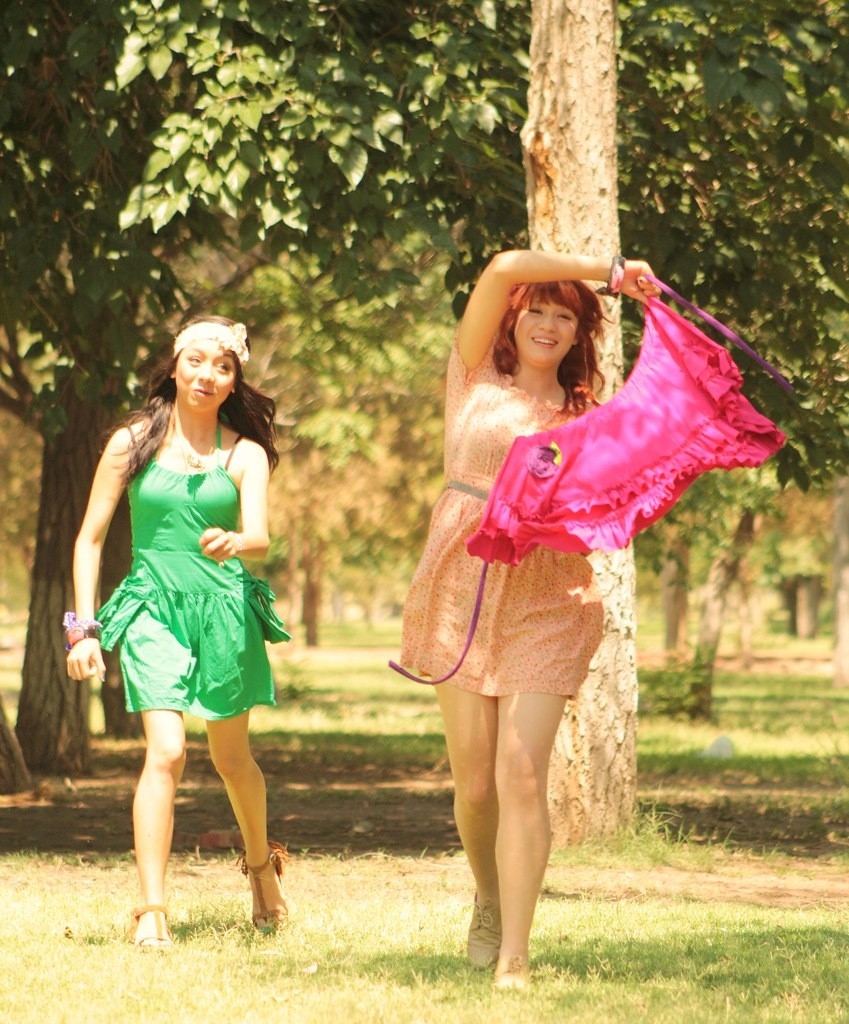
[
  {"xmin": 467, "ymin": 893, "xmax": 502, "ymax": 970},
  {"xmin": 495, "ymin": 956, "xmax": 530, "ymax": 994}
]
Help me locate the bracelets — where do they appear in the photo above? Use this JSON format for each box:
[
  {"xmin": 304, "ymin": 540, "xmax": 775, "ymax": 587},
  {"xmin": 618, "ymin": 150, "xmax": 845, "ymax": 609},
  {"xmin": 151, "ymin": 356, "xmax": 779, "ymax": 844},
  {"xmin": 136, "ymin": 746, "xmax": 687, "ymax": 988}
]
[
  {"xmin": 596, "ymin": 254, "xmax": 625, "ymax": 300},
  {"xmin": 63, "ymin": 612, "xmax": 104, "ymax": 644}
]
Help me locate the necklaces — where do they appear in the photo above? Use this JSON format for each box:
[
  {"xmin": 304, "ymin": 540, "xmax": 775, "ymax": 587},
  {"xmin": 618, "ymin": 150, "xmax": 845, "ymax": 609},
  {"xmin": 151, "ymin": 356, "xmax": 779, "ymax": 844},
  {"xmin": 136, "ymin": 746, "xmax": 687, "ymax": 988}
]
[{"xmin": 176, "ymin": 430, "xmax": 214, "ymax": 469}]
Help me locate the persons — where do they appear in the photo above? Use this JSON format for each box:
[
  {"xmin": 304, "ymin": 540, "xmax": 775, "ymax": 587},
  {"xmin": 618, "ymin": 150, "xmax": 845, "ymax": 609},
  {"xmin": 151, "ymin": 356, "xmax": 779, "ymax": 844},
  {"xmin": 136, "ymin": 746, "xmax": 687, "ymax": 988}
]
[
  {"xmin": 388, "ymin": 249, "xmax": 663, "ymax": 995},
  {"xmin": 64, "ymin": 315, "xmax": 288, "ymax": 954}
]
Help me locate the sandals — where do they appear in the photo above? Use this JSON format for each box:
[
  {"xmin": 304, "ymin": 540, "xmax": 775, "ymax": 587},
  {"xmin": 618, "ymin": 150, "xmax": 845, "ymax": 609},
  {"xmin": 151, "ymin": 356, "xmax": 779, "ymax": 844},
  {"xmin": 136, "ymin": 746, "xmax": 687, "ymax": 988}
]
[
  {"xmin": 240, "ymin": 841, "xmax": 288, "ymax": 928},
  {"xmin": 133, "ymin": 905, "xmax": 174, "ymax": 950}
]
